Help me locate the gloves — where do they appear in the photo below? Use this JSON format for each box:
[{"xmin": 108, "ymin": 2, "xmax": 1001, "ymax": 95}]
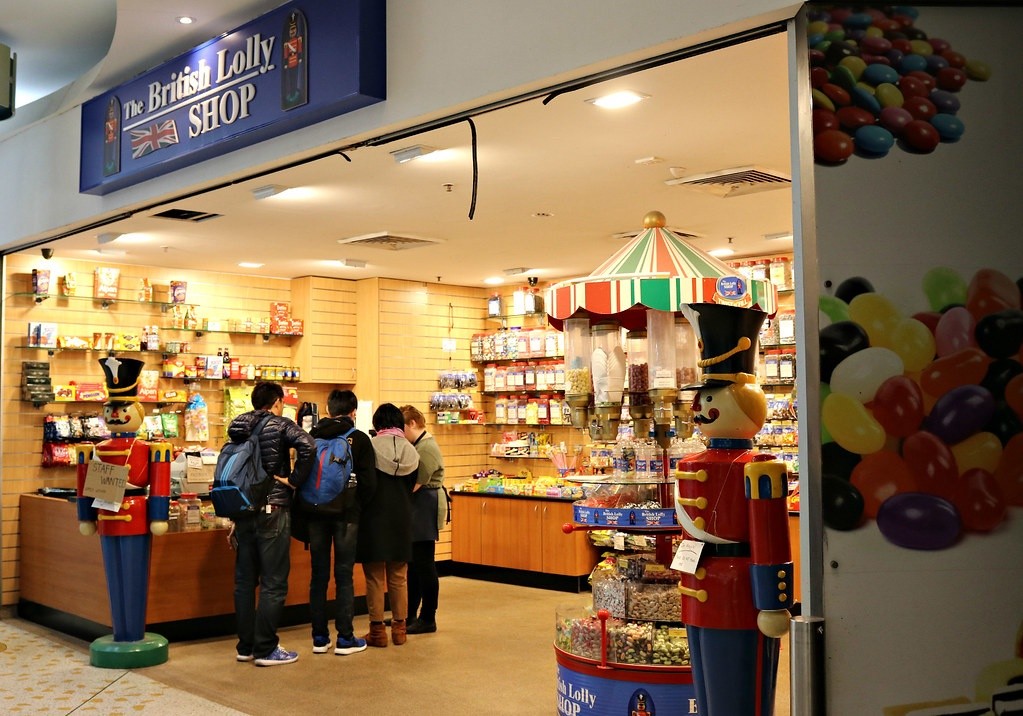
[{"xmin": 297, "ymin": 427, "xmax": 359, "ymax": 513}]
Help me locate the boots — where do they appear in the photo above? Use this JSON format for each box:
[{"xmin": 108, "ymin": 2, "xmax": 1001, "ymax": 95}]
[
  {"xmin": 361, "ymin": 623, "xmax": 388, "ymax": 646},
  {"xmin": 391, "ymin": 618, "xmax": 406, "ymax": 646}
]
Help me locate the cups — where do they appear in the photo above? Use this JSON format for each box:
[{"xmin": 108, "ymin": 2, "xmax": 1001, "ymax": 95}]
[
  {"xmin": 217, "ymin": 348, "xmax": 222, "ymax": 356},
  {"xmin": 223, "ymin": 348, "xmax": 230, "ymax": 378}
]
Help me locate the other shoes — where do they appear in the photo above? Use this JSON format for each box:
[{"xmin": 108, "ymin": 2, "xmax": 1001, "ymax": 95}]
[
  {"xmin": 384, "ymin": 616, "xmax": 418, "ymax": 626},
  {"xmin": 406, "ymin": 620, "xmax": 436, "ymax": 634}
]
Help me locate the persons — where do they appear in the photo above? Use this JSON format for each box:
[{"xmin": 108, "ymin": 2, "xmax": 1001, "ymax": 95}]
[
  {"xmin": 292, "ymin": 389, "xmax": 376, "ymax": 655},
  {"xmin": 226, "ymin": 381, "xmax": 318, "ymax": 666},
  {"xmin": 354, "ymin": 403, "xmax": 418, "ymax": 647},
  {"xmin": 75, "ymin": 357, "xmax": 174, "ymax": 642},
  {"xmin": 384, "ymin": 406, "xmax": 452, "ymax": 635},
  {"xmin": 670, "ymin": 303, "xmax": 795, "ymax": 716}
]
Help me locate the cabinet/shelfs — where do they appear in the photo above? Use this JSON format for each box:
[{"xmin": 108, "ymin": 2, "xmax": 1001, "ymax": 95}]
[
  {"xmin": 14, "ymin": 292, "xmax": 303, "ymax": 404},
  {"xmin": 428, "ymin": 289, "xmax": 800, "ymax": 716}
]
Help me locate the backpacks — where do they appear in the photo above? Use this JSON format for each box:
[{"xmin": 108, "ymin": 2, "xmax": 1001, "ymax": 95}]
[{"xmin": 211, "ymin": 414, "xmax": 289, "ymax": 517}]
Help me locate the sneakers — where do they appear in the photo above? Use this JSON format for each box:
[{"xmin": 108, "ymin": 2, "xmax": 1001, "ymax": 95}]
[
  {"xmin": 334, "ymin": 636, "xmax": 368, "ymax": 655},
  {"xmin": 255, "ymin": 644, "xmax": 298, "ymax": 666},
  {"xmin": 313, "ymin": 635, "xmax": 332, "ymax": 653},
  {"xmin": 237, "ymin": 653, "xmax": 254, "ymax": 661}
]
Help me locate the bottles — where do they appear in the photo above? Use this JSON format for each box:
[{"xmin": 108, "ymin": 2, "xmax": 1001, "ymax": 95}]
[
  {"xmin": 727, "ymin": 257, "xmax": 798, "ymax": 473},
  {"xmin": 140, "ymin": 328, "xmax": 147, "ymax": 352},
  {"xmin": 591, "ymin": 421, "xmax": 706, "ymax": 479},
  {"xmin": 169, "ymin": 493, "xmax": 203, "ymax": 524},
  {"xmin": 470, "ymin": 327, "xmax": 570, "ymax": 424}
]
